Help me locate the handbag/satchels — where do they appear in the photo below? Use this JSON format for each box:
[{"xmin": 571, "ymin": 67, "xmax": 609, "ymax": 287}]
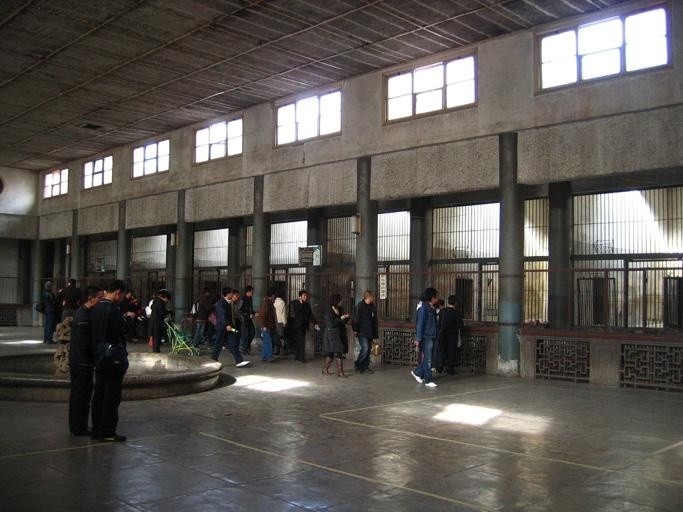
[
  {"xmin": 277, "ymin": 336, "xmax": 289, "ymax": 349},
  {"xmin": 190, "ymin": 300, "xmax": 200, "ymax": 318},
  {"xmin": 370, "ymin": 340, "xmax": 381, "ymax": 357},
  {"xmin": 97, "ymin": 343, "xmax": 127, "ymax": 375},
  {"xmin": 439, "ymin": 328, "xmax": 447, "ymax": 352},
  {"xmin": 36, "ymin": 303, "xmax": 45, "ymax": 313},
  {"xmin": 148, "ymin": 335, "xmax": 154, "ymax": 346},
  {"xmin": 208, "ymin": 313, "xmax": 218, "ymax": 326}
]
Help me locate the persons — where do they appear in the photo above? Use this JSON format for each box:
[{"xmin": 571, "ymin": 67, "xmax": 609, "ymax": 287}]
[
  {"xmin": 145, "ymin": 288, "xmax": 172, "ymax": 352},
  {"xmin": 69, "ymin": 285, "xmax": 103, "ymax": 436},
  {"xmin": 320, "ymin": 290, "xmax": 379, "ymax": 379},
  {"xmin": 191, "ymin": 285, "xmax": 262, "ymax": 367},
  {"xmin": 258, "ymin": 287, "xmax": 320, "ymax": 363},
  {"xmin": 79, "ymin": 279, "xmax": 129, "ymax": 441},
  {"xmin": 42, "ymin": 279, "xmax": 145, "ymax": 345},
  {"xmin": 410, "ymin": 287, "xmax": 465, "ymax": 387}
]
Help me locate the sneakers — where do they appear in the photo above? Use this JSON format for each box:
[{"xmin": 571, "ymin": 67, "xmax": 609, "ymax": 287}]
[
  {"xmin": 410, "ymin": 370, "xmax": 424, "ymax": 384},
  {"xmin": 102, "ymin": 436, "xmax": 126, "ymax": 442},
  {"xmin": 236, "ymin": 360, "xmax": 250, "ymax": 367},
  {"xmin": 425, "ymin": 382, "xmax": 437, "ymax": 388},
  {"xmin": 360, "ymin": 367, "xmax": 374, "ymax": 374},
  {"xmin": 43, "ymin": 339, "xmax": 57, "ymax": 344},
  {"xmin": 73, "ymin": 427, "xmax": 91, "ymax": 436}
]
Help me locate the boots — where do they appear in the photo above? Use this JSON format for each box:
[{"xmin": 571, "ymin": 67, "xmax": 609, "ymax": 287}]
[
  {"xmin": 335, "ymin": 357, "xmax": 345, "ymax": 377},
  {"xmin": 322, "ymin": 357, "xmax": 332, "ymax": 376}
]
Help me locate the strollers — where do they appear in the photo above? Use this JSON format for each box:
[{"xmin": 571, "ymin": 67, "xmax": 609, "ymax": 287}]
[{"xmin": 164, "ymin": 317, "xmax": 200, "ymax": 357}]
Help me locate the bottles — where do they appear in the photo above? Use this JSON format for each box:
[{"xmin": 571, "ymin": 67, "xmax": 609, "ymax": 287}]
[
  {"xmin": 415, "ymin": 342, "xmax": 419, "ymax": 353},
  {"xmin": 229, "ymin": 328, "xmax": 239, "ymax": 333}
]
